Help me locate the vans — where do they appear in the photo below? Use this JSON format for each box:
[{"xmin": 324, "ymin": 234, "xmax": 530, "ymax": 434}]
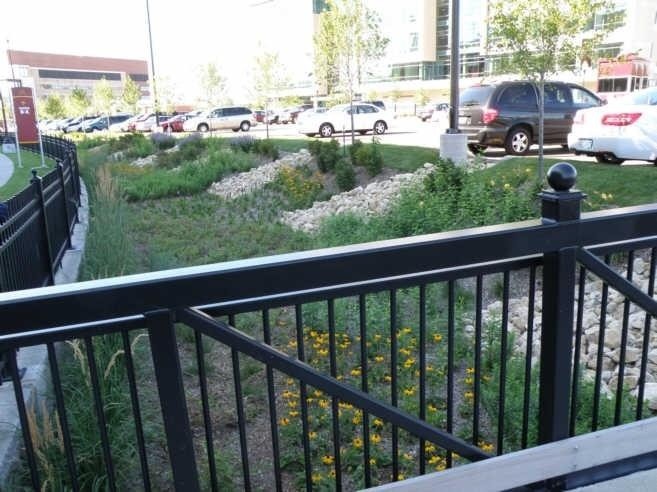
[{"xmin": 450, "ymin": 80, "xmax": 607, "ymax": 154}]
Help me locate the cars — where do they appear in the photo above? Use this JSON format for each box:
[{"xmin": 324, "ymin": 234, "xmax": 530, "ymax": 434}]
[
  {"xmin": 299, "ymin": 105, "xmax": 390, "ymax": 137},
  {"xmin": 567, "ymin": 85, "xmax": 657, "ymax": 165},
  {"xmin": 416, "ymin": 102, "xmax": 449, "ymax": 122},
  {"xmin": 37, "ymin": 106, "xmax": 326, "ymax": 131}
]
